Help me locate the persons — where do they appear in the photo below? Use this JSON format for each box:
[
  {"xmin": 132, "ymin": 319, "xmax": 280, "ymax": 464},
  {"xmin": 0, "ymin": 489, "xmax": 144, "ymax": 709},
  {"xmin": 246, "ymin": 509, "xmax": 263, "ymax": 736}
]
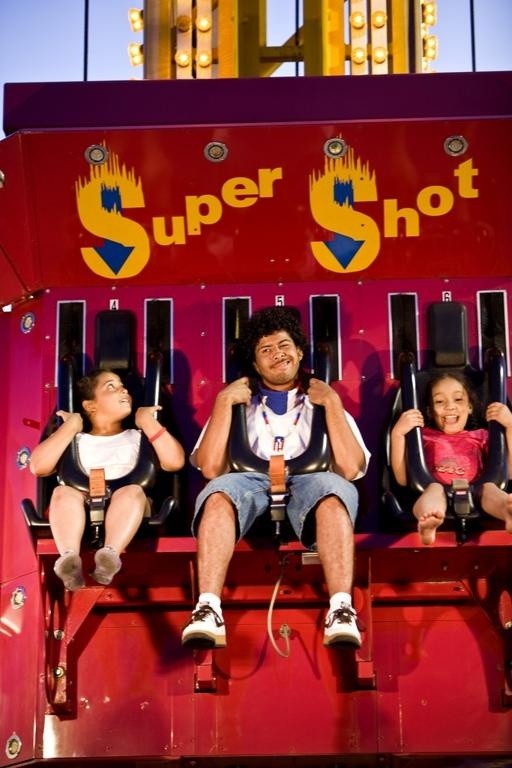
[
  {"xmin": 390, "ymin": 366, "xmax": 512, "ymax": 545},
  {"xmin": 28, "ymin": 370, "xmax": 186, "ymax": 591},
  {"xmin": 182, "ymin": 306, "xmax": 372, "ymax": 652}
]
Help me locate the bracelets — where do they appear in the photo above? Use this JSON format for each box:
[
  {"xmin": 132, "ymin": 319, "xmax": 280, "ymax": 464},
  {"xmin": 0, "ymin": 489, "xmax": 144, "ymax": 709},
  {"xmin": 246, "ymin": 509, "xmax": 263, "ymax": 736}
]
[{"xmin": 147, "ymin": 426, "xmax": 166, "ymax": 444}]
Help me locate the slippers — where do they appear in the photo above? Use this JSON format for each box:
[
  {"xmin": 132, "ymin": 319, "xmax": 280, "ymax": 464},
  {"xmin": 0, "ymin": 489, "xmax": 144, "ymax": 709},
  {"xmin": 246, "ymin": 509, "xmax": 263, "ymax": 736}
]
[{"xmin": 54, "ymin": 549, "xmax": 121, "ymax": 590}]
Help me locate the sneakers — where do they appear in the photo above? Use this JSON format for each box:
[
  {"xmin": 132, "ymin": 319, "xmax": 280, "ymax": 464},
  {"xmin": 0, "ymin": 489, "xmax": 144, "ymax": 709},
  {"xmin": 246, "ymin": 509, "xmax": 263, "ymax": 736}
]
[
  {"xmin": 182, "ymin": 602, "xmax": 227, "ymax": 648},
  {"xmin": 324, "ymin": 603, "xmax": 362, "ymax": 647}
]
